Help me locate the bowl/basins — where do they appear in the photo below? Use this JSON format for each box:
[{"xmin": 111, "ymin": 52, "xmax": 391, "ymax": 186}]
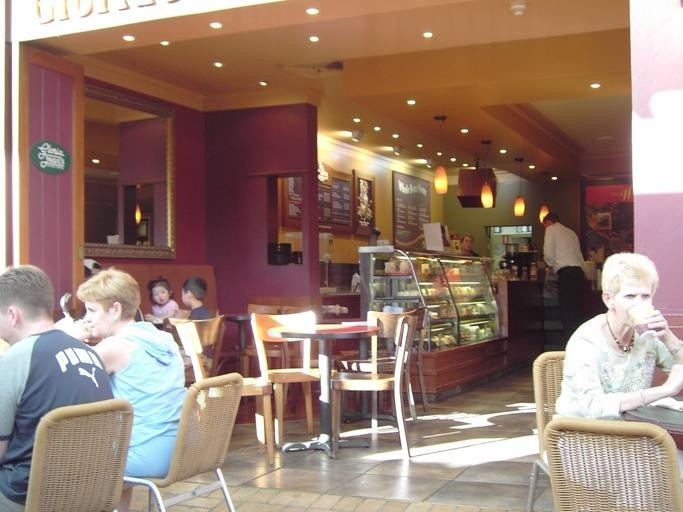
[{"xmin": 272, "ymin": 242, "xmax": 291, "ymax": 264}]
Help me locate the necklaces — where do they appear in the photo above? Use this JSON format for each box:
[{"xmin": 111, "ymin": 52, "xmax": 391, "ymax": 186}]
[{"xmin": 601, "ymin": 316, "xmax": 637, "ymax": 352}]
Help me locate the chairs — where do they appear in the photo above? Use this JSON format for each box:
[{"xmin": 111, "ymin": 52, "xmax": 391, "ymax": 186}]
[
  {"xmin": 527, "ymin": 349, "xmax": 565, "ymax": 512},
  {"xmin": 136, "ymin": 304, "xmax": 320, "ymax": 467},
  {"xmin": 122, "ymin": 372, "xmax": 245, "ymax": 511},
  {"xmin": 331, "ymin": 305, "xmax": 429, "ymax": 460},
  {"xmin": 544, "ymin": 417, "xmax": 683, "ymax": 512},
  {"xmin": 20, "ymin": 398, "xmax": 132, "ymax": 511}
]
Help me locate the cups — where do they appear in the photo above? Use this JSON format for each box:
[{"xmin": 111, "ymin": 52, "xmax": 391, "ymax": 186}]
[
  {"xmin": 382, "ymin": 305, "xmax": 403, "ymax": 316},
  {"xmin": 290, "ymin": 250, "xmax": 301, "ymax": 264},
  {"xmin": 384, "ymin": 260, "xmax": 459, "ymax": 282},
  {"xmin": 627, "ymin": 302, "xmax": 657, "ymax": 341},
  {"xmin": 510, "ymin": 260, "xmax": 538, "ymax": 282}
]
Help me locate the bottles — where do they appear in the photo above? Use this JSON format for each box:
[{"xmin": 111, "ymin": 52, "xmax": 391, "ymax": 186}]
[{"xmin": 350, "ymin": 272, "xmax": 360, "ymax": 292}]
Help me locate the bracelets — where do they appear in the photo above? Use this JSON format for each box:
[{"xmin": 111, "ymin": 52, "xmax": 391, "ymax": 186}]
[
  {"xmin": 666, "ymin": 338, "xmax": 681, "ymax": 355},
  {"xmin": 638, "ymin": 389, "xmax": 647, "ymax": 407}
]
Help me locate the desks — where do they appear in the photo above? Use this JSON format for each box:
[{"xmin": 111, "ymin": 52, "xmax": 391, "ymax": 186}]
[{"xmin": 268, "ymin": 321, "xmax": 379, "ymax": 460}]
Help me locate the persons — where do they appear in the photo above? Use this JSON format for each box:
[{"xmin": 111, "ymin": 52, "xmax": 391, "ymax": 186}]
[
  {"xmin": 542, "ymin": 213, "xmax": 583, "ymax": 339},
  {"xmin": 144, "ymin": 275, "xmax": 178, "ymax": 325},
  {"xmin": 544, "ymin": 250, "xmax": 681, "ymax": 508},
  {"xmin": 0, "ymin": 264, "xmax": 112, "ymax": 509},
  {"xmin": 64, "ymin": 266, "xmax": 187, "ymax": 507},
  {"xmin": 459, "ymin": 233, "xmax": 478, "ymax": 255},
  {"xmin": 181, "ymin": 275, "xmax": 214, "ymax": 368}
]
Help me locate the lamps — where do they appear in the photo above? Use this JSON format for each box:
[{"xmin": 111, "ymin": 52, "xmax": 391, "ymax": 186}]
[
  {"xmin": 433, "ymin": 115, "xmax": 449, "ymax": 195},
  {"xmin": 538, "ymin": 169, "xmax": 549, "ymax": 224},
  {"xmin": 479, "ymin": 139, "xmax": 493, "ymax": 209},
  {"xmin": 513, "ymin": 156, "xmax": 527, "ymax": 216}
]
